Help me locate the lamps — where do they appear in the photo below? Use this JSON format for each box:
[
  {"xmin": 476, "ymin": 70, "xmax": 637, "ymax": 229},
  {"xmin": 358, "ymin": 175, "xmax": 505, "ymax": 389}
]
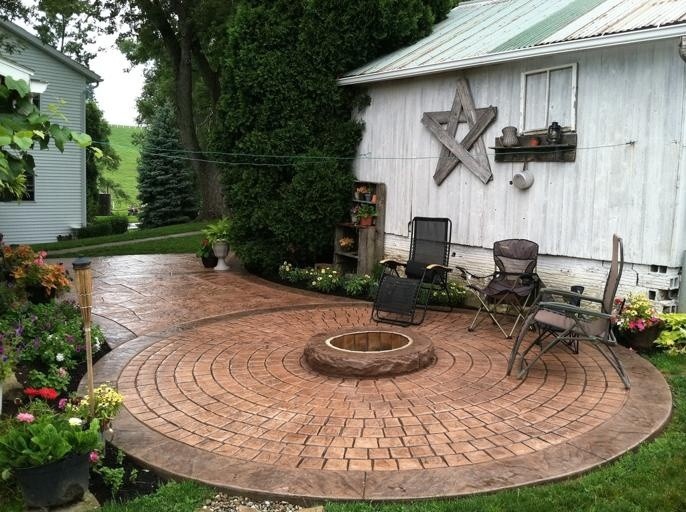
[{"xmin": 546, "ymin": 121, "xmax": 561, "ymax": 145}]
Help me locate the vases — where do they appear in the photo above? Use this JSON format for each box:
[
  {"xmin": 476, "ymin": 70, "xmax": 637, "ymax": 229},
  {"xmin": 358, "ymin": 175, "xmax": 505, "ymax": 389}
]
[
  {"xmin": 626, "ymin": 334, "xmax": 656, "ymax": 347},
  {"xmin": 12, "ymin": 449, "xmax": 91, "ymax": 509}
]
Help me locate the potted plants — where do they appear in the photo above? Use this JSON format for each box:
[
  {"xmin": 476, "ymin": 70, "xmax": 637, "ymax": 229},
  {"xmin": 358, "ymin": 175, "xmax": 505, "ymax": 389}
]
[
  {"xmin": 200, "ymin": 216, "xmax": 236, "ymax": 271},
  {"xmin": 527, "ymin": 132, "xmax": 542, "ymax": 146},
  {"xmin": 340, "ymin": 186, "xmax": 379, "ymax": 257}
]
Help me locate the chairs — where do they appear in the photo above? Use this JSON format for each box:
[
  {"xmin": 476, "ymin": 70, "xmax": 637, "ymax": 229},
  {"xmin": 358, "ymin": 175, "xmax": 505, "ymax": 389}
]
[
  {"xmin": 455, "ymin": 238, "xmax": 539, "ymax": 339},
  {"xmin": 507, "ymin": 234, "xmax": 633, "ymax": 390},
  {"xmin": 370, "ymin": 212, "xmax": 453, "ymax": 329}
]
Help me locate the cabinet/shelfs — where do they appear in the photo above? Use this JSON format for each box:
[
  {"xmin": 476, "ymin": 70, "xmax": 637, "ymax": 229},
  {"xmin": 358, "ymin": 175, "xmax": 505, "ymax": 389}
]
[{"xmin": 331, "ymin": 179, "xmax": 386, "ymax": 279}]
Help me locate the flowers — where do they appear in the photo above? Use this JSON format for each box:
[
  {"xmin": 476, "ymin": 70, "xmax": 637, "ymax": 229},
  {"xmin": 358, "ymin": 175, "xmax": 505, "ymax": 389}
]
[
  {"xmin": 0, "ymin": 383, "xmax": 124, "ymax": 466},
  {"xmin": 610, "ymin": 292, "xmax": 658, "ymax": 336}
]
